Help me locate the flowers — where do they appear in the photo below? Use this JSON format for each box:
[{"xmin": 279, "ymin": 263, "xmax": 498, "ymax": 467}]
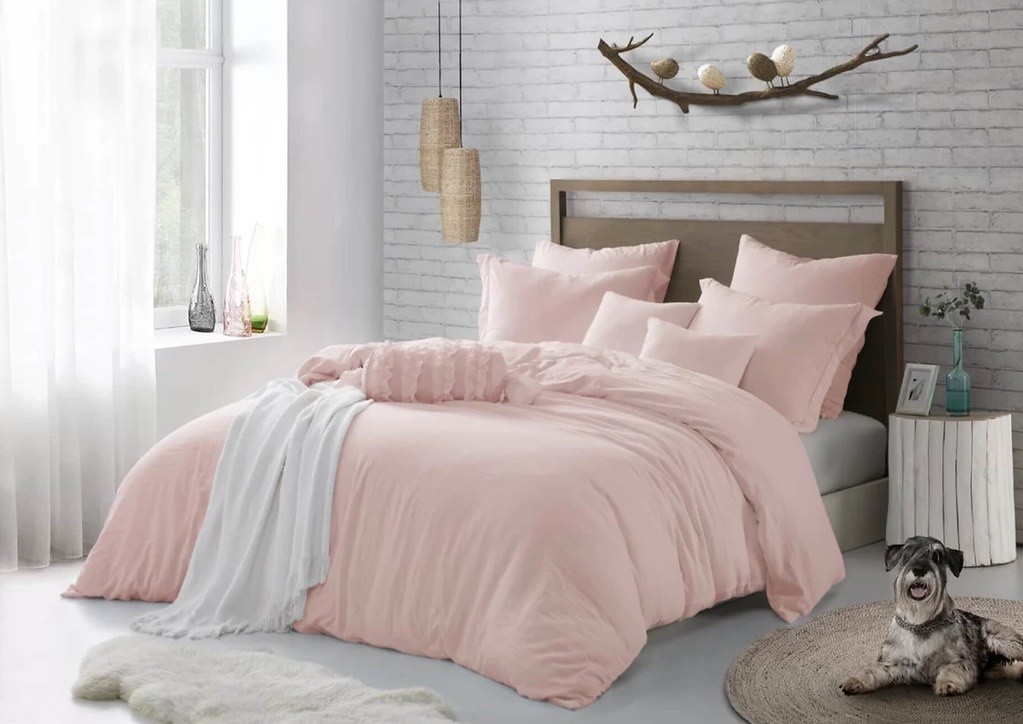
[{"xmin": 919, "ymin": 278, "xmax": 987, "ymax": 370}]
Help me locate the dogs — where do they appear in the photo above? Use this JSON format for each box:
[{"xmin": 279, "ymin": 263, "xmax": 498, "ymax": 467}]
[{"xmin": 839, "ymin": 536, "xmax": 1023, "ymax": 698}]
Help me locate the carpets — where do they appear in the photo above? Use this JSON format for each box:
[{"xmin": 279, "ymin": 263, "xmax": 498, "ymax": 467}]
[
  {"xmin": 722, "ymin": 593, "xmax": 1023, "ymax": 724},
  {"xmin": 69, "ymin": 632, "xmax": 461, "ymax": 723}
]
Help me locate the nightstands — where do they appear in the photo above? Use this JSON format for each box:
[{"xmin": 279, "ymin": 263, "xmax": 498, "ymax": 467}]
[{"xmin": 885, "ymin": 409, "xmax": 1018, "ymax": 568}]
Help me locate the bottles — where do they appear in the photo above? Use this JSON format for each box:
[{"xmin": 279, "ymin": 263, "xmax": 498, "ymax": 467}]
[
  {"xmin": 247, "ymin": 282, "xmax": 268, "ymax": 334},
  {"xmin": 944, "ymin": 328, "xmax": 970, "ymax": 417},
  {"xmin": 220, "ymin": 235, "xmax": 252, "ymax": 336},
  {"xmin": 187, "ymin": 241, "xmax": 215, "ymax": 332}
]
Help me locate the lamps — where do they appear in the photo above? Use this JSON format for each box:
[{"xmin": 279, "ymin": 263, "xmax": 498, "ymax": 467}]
[
  {"xmin": 418, "ymin": 0, "xmax": 462, "ymax": 192},
  {"xmin": 440, "ymin": 0, "xmax": 483, "ymax": 248}
]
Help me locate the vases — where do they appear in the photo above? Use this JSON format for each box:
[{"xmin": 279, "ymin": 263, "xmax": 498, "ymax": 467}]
[
  {"xmin": 944, "ymin": 327, "xmax": 971, "ymax": 417},
  {"xmin": 187, "ymin": 242, "xmax": 217, "ymax": 332},
  {"xmin": 223, "ymin": 233, "xmax": 255, "ymax": 338},
  {"xmin": 246, "ymin": 221, "xmax": 270, "ymax": 333}
]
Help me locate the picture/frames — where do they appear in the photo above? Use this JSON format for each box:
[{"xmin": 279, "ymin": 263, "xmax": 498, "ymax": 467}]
[{"xmin": 894, "ymin": 361, "xmax": 940, "ymax": 417}]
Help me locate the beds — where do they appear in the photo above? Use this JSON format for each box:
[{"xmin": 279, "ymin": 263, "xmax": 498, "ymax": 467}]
[{"xmin": 58, "ymin": 399, "xmax": 907, "ymax": 712}]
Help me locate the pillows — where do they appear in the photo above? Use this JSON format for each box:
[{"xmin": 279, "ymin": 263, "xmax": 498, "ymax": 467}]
[
  {"xmin": 528, "ymin": 238, "xmax": 682, "ymax": 305},
  {"xmin": 476, "ymin": 252, "xmax": 670, "ymax": 345},
  {"xmin": 684, "ymin": 277, "xmax": 885, "ymax": 435},
  {"xmin": 577, "ymin": 288, "xmax": 702, "ymax": 360},
  {"xmin": 637, "ymin": 315, "xmax": 763, "ymax": 389},
  {"xmin": 727, "ymin": 232, "xmax": 900, "ymax": 420}
]
